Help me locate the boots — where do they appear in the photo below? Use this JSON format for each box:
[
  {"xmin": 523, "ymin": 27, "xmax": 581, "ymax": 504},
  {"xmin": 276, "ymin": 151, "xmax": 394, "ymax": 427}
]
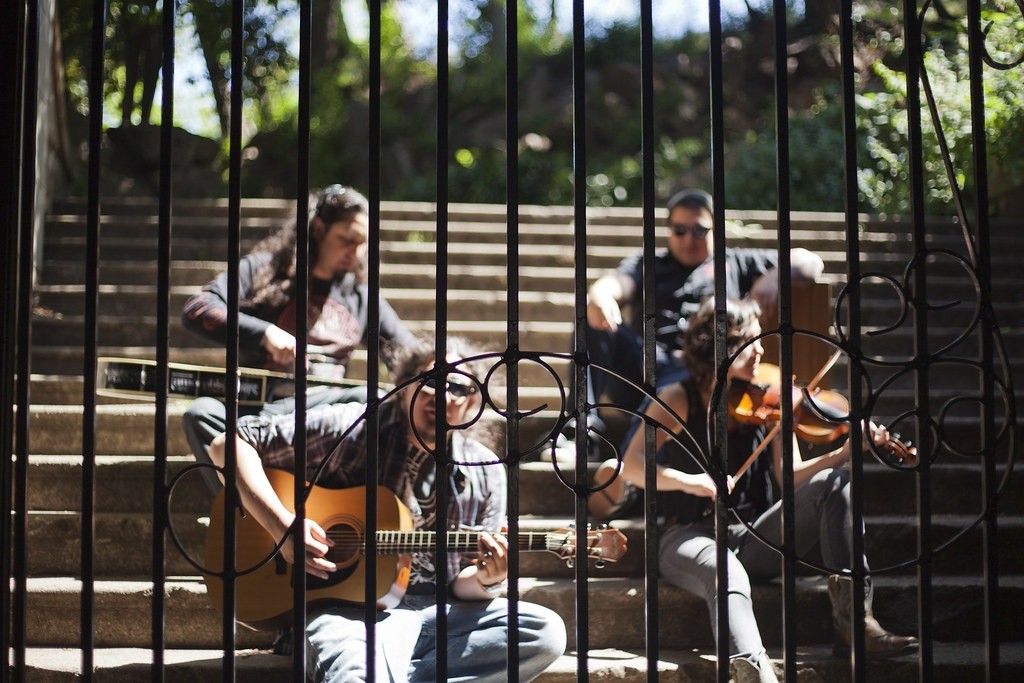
[
  {"xmin": 828, "ymin": 575, "xmax": 919, "ymax": 659},
  {"xmin": 728, "ymin": 653, "xmax": 779, "ymax": 683}
]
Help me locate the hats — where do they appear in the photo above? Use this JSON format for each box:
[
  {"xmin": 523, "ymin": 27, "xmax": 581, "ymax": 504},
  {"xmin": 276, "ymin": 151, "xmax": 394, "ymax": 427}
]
[
  {"xmin": 664, "ymin": 187, "xmax": 713, "ymax": 214},
  {"xmin": 316, "ymin": 183, "xmax": 369, "ymax": 211}
]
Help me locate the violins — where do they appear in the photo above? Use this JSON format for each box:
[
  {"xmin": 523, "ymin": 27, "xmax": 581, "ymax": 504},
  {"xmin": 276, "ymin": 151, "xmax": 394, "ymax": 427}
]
[{"xmin": 724, "ymin": 361, "xmax": 919, "ymax": 466}]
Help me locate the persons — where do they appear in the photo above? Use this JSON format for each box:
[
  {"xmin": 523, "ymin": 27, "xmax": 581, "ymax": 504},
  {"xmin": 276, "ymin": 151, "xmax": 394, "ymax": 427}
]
[
  {"xmin": 210, "ymin": 335, "xmax": 568, "ymax": 683},
  {"xmin": 181, "ymin": 182, "xmax": 417, "ymax": 499},
  {"xmin": 623, "ymin": 296, "xmax": 920, "ymax": 683},
  {"xmin": 569, "ymin": 188, "xmax": 824, "ymax": 518}
]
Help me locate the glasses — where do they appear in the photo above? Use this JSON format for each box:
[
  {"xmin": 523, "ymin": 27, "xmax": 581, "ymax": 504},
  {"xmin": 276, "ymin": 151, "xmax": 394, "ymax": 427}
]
[
  {"xmin": 671, "ymin": 224, "xmax": 711, "ymax": 238},
  {"xmin": 417, "ymin": 371, "xmax": 479, "ymax": 397}
]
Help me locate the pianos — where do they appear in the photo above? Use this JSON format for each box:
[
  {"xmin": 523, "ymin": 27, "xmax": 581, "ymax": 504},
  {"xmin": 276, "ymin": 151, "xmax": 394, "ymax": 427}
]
[{"xmin": 89, "ymin": 355, "xmax": 409, "ymax": 410}]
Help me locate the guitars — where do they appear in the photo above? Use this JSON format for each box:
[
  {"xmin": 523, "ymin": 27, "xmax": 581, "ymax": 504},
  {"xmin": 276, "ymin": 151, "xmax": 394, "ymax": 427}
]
[{"xmin": 203, "ymin": 466, "xmax": 630, "ymax": 634}]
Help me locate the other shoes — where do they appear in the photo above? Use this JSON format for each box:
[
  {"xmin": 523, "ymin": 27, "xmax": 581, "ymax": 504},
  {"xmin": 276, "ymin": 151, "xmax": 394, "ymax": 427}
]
[
  {"xmin": 539, "ymin": 432, "xmax": 602, "ymax": 462},
  {"xmin": 586, "ymin": 458, "xmax": 633, "ymax": 519}
]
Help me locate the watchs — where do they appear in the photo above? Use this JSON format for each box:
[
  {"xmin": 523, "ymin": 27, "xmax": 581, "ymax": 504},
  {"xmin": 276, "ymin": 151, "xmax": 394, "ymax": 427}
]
[{"xmin": 477, "ymin": 579, "xmax": 506, "ymax": 593}]
